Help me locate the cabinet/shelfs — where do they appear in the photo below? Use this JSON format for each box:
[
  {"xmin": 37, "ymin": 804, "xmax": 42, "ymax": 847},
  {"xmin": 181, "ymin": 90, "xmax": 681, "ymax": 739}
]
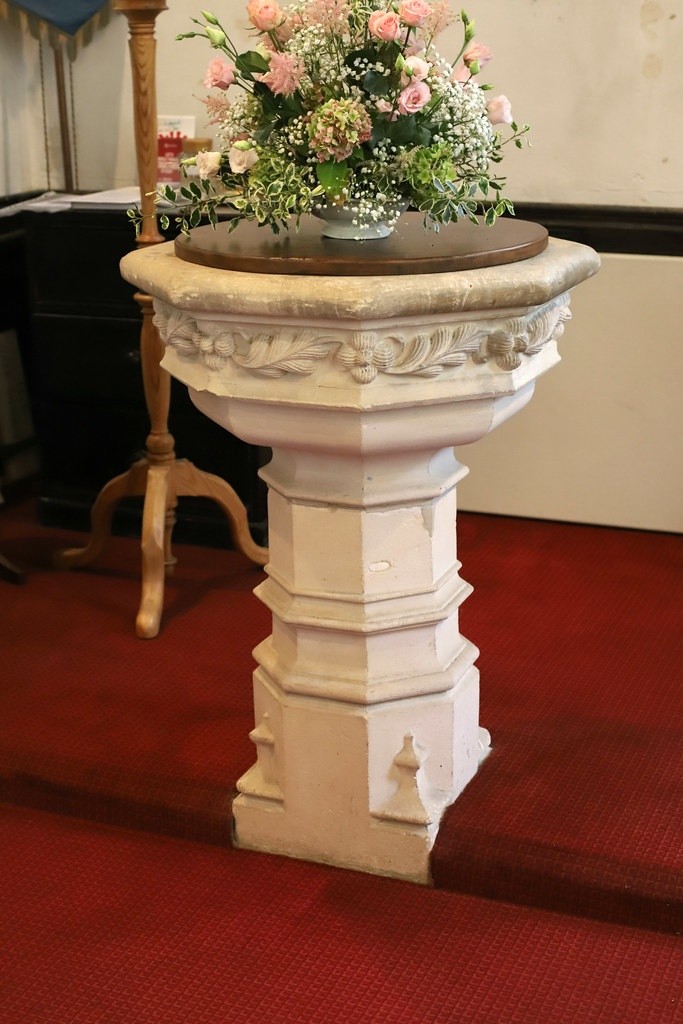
[{"xmin": 25, "ymin": 204, "xmax": 267, "ymax": 546}]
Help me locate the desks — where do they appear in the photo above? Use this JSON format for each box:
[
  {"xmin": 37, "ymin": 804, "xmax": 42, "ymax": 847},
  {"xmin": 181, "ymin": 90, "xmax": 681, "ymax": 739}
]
[{"xmin": 120, "ymin": 208, "xmax": 602, "ymax": 887}]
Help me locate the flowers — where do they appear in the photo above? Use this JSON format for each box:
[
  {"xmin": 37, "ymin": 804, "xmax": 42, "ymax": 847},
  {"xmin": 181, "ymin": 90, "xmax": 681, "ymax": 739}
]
[{"xmin": 127, "ymin": 0, "xmax": 527, "ymax": 241}]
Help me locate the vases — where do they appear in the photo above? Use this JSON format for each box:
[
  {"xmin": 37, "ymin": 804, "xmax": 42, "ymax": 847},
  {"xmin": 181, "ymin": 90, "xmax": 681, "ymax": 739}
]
[{"xmin": 308, "ymin": 195, "xmax": 410, "ymax": 240}]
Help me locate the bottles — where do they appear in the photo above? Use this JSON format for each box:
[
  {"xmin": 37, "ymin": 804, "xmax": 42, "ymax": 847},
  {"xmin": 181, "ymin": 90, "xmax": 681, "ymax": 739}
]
[{"xmin": 180, "ymin": 138, "xmax": 215, "ymax": 198}]
